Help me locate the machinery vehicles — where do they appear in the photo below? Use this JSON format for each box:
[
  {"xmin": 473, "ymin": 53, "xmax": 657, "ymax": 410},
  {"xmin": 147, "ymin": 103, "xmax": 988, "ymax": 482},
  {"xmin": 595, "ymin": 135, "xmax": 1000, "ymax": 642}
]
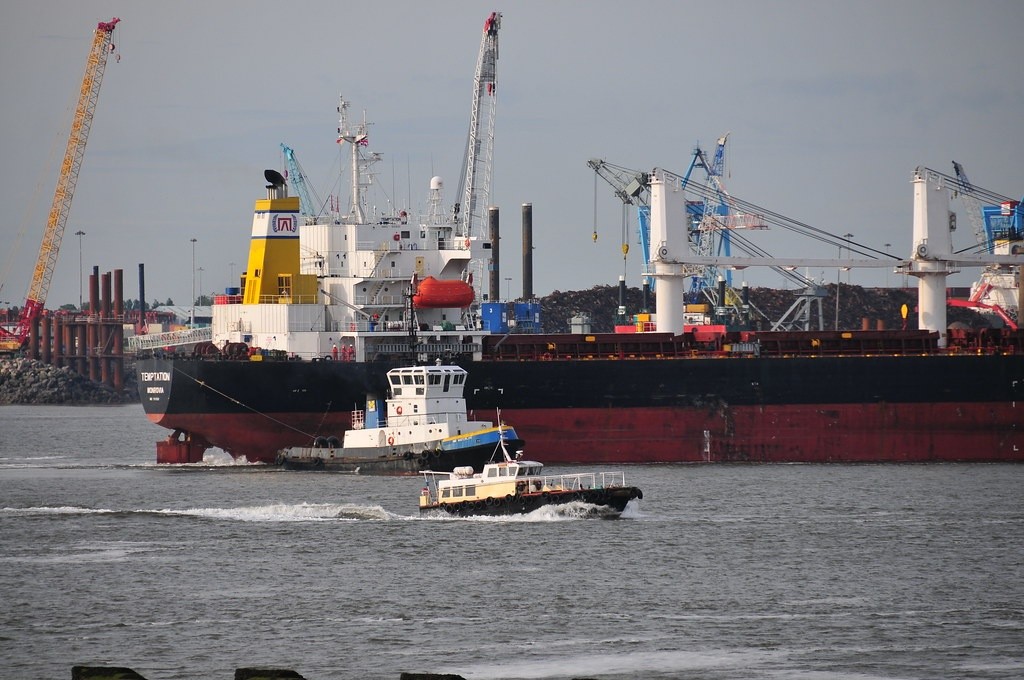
[{"xmin": 0, "ymin": 16, "xmax": 123, "ymax": 360}]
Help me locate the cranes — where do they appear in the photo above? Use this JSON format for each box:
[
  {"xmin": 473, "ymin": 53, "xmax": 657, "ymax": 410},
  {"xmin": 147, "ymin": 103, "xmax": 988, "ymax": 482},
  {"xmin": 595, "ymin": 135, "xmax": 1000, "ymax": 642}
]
[
  {"xmin": 585, "ymin": 131, "xmax": 1023, "ymax": 350},
  {"xmin": 452, "ymin": 10, "xmax": 505, "ymax": 325}
]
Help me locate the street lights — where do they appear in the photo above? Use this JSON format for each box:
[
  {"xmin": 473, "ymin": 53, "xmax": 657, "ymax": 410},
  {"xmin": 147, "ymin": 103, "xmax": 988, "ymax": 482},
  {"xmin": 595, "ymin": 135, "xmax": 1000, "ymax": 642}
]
[
  {"xmin": 884, "ymin": 243, "xmax": 892, "ymax": 287},
  {"xmin": 75, "ymin": 230, "xmax": 87, "ymax": 310},
  {"xmin": 197, "ymin": 266, "xmax": 204, "ymax": 306},
  {"xmin": 229, "ymin": 262, "xmax": 233, "ymax": 288},
  {"xmin": 844, "ymin": 233, "xmax": 856, "ymax": 285},
  {"xmin": 190, "ymin": 238, "xmax": 198, "ymax": 330}
]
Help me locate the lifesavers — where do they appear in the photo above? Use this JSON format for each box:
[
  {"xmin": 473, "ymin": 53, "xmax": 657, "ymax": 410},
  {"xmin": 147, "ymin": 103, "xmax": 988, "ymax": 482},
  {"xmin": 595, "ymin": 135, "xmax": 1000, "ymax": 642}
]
[
  {"xmin": 551, "ymin": 495, "xmax": 560, "ymax": 503},
  {"xmin": 541, "ymin": 491, "xmax": 549, "ymax": 499},
  {"xmin": 421, "ymin": 451, "xmax": 429, "ymax": 459},
  {"xmin": 388, "ymin": 437, "xmax": 394, "ymax": 445},
  {"xmin": 433, "ymin": 449, "xmax": 442, "ymax": 457},
  {"xmin": 461, "ymin": 500, "xmax": 468, "ymax": 509},
  {"xmin": 396, "ymin": 406, "xmax": 403, "ymax": 415},
  {"xmin": 446, "ymin": 504, "xmax": 454, "ymax": 514},
  {"xmin": 512, "ymin": 495, "xmax": 518, "ymax": 503},
  {"xmin": 526, "ymin": 496, "xmax": 534, "ymax": 505},
  {"xmin": 504, "ymin": 494, "xmax": 512, "ymax": 503},
  {"xmin": 493, "ymin": 498, "xmax": 501, "ymax": 506},
  {"xmin": 464, "ymin": 240, "xmax": 470, "ymax": 247},
  {"xmin": 573, "ymin": 489, "xmax": 614, "ymax": 502},
  {"xmin": 403, "ymin": 451, "xmax": 412, "ymax": 460},
  {"xmin": 485, "ymin": 496, "xmax": 493, "ymax": 505},
  {"xmin": 475, "ymin": 501, "xmax": 483, "ymax": 509},
  {"xmin": 453, "ymin": 502, "xmax": 461, "ymax": 512},
  {"xmin": 468, "ymin": 502, "xmax": 476, "ymax": 511},
  {"xmin": 518, "ymin": 496, "xmax": 525, "ymax": 505}
]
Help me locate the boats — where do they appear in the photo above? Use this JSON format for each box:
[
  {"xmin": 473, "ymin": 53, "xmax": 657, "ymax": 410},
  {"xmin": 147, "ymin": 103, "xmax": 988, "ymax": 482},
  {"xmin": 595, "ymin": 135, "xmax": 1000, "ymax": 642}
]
[
  {"xmin": 417, "ymin": 405, "xmax": 643, "ymax": 522},
  {"xmin": 272, "ymin": 282, "xmax": 526, "ymax": 478},
  {"xmin": 136, "ymin": 90, "xmax": 1023, "ymax": 472}
]
